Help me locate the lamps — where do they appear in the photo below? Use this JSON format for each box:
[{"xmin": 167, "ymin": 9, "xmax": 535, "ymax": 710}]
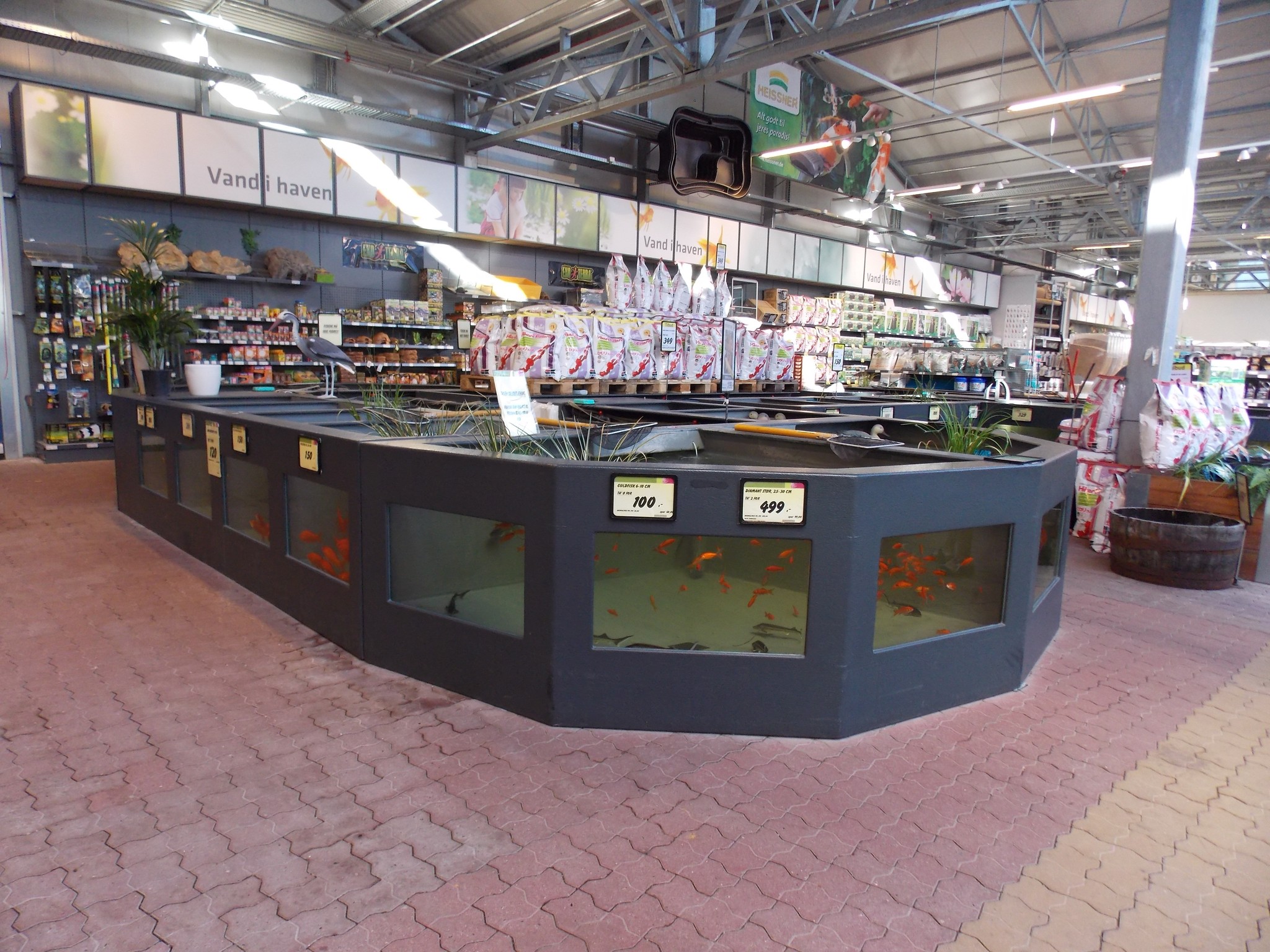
[
  {"xmin": 979, "ymin": 183, "xmax": 985, "ymax": 187},
  {"xmin": 1002, "ymin": 179, "xmax": 1010, "ymax": 185},
  {"xmin": 996, "ymin": 181, "xmax": 1004, "ymax": 190},
  {"xmin": 1237, "ymin": 150, "xmax": 1251, "ymax": 162},
  {"xmin": 891, "ymin": 183, "xmax": 961, "ymax": 199},
  {"xmin": 758, "ymin": 140, "xmax": 833, "ymax": 160},
  {"xmin": 842, "ymin": 131, "xmax": 891, "ymax": 150},
  {"xmin": 1247, "ymin": 147, "xmax": 1259, "ymax": 153},
  {"xmin": 971, "ymin": 184, "xmax": 981, "ymax": 193},
  {"xmin": 1006, "ymin": 83, "xmax": 1124, "ymax": 114},
  {"xmin": 1117, "ymin": 151, "xmax": 1221, "ymax": 169}
]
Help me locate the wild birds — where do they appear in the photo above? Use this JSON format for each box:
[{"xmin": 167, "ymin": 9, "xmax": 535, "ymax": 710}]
[{"xmin": 269, "ymin": 310, "xmax": 355, "ymax": 400}]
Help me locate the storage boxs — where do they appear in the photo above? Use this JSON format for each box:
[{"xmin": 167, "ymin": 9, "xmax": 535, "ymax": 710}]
[
  {"xmin": 826, "ymin": 288, "xmax": 978, "ymax": 392},
  {"xmin": 490, "ymin": 275, "xmax": 542, "ymax": 299},
  {"xmin": 43, "ymin": 419, "xmax": 69, "ymax": 444},
  {"xmin": 763, "ymin": 288, "xmax": 788, "ymax": 300},
  {"xmin": 102, "ymin": 422, "xmax": 113, "ymax": 441},
  {"xmin": 765, "ymin": 300, "xmax": 787, "ymax": 314},
  {"xmin": 744, "ymin": 299, "xmax": 780, "ymax": 323},
  {"xmin": 66, "ymin": 421, "xmax": 103, "ymax": 443},
  {"xmin": 775, "ymin": 314, "xmax": 786, "ymax": 324},
  {"xmin": 188, "ymin": 266, "xmax": 477, "ymax": 389}
]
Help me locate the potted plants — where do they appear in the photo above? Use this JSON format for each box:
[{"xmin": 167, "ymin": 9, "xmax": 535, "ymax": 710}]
[{"xmin": 87, "ymin": 219, "xmax": 199, "ymax": 400}]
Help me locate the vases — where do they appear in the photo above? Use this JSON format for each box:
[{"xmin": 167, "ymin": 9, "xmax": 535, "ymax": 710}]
[{"xmin": 183, "ymin": 365, "xmax": 223, "ymax": 397}]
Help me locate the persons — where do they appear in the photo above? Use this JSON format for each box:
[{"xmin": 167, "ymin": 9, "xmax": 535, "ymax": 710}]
[
  {"xmin": 862, "ymin": 102, "xmax": 892, "ymax": 124},
  {"xmin": 479, "ymin": 174, "xmax": 528, "ymax": 240}
]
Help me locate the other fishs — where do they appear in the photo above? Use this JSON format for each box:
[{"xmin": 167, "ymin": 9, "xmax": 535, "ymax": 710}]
[
  {"xmin": 445, "ymin": 589, "xmax": 470, "ymax": 616},
  {"xmin": 885, "ymin": 596, "xmax": 923, "ymax": 618},
  {"xmin": 592, "ymin": 622, "xmax": 804, "ymax": 655}
]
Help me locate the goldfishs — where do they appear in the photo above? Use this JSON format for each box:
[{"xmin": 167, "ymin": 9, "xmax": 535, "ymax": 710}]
[
  {"xmin": 249, "ymin": 512, "xmax": 270, "ymax": 541},
  {"xmin": 494, "ymin": 521, "xmax": 525, "ymax": 552},
  {"xmin": 595, "ymin": 530, "xmax": 803, "ymax": 620},
  {"xmin": 300, "ymin": 507, "xmax": 350, "ymax": 582},
  {"xmin": 876, "ymin": 534, "xmax": 985, "ymax": 602},
  {"xmin": 936, "ymin": 629, "xmax": 952, "ymax": 636}
]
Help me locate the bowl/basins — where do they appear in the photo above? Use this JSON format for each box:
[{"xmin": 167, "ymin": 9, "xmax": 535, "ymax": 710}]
[
  {"xmin": 184, "ymin": 363, "xmax": 223, "ymax": 395},
  {"xmin": 1106, "ymin": 506, "xmax": 1246, "ymax": 589}
]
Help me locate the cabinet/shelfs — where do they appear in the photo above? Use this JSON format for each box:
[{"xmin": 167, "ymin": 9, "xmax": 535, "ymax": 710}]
[{"xmin": 836, "ymin": 324, "xmax": 1031, "ymax": 396}]
